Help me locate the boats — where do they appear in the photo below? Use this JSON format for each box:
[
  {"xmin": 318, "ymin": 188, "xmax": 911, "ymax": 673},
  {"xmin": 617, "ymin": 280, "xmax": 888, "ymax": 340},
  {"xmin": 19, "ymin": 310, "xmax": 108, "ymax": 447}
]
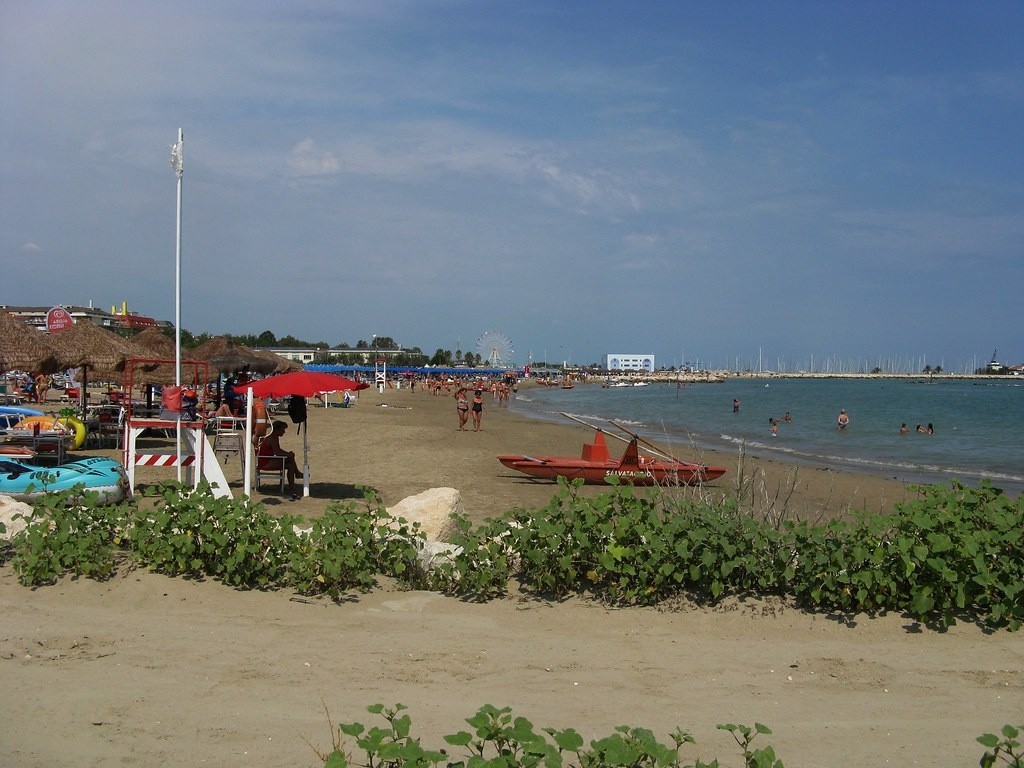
[
  {"xmin": 560, "ymin": 384, "xmax": 575, "ymax": 389},
  {"xmin": 0, "ymin": 406, "xmax": 128, "ymax": 507},
  {"xmin": 535, "ymin": 377, "xmax": 558, "ymax": 385},
  {"xmin": 606, "ymin": 378, "xmax": 651, "ymax": 388},
  {"xmin": 497, "ymin": 429, "xmax": 727, "ymax": 486}
]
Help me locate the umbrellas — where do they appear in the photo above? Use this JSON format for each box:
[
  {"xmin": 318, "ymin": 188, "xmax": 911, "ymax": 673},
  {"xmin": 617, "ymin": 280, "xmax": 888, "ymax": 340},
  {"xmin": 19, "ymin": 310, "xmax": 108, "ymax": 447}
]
[
  {"xmin": 191, "ymin": 336, "xmax": 279, "ymax": 373},
  {"xmin": 73, "ymin": 325, "xmax": 220, "ymax": 385},
  {"xmin": 50, "ymin": 318, "xmax": 163, "ymax": 372},
  {"xmin": 233, "ymin": 371, "xmax": 370, "ymax": 496},
  {"xmin": 0, "ymin": 309, "xmax": 84, "ymax": 371},
  {"xmin": 259, "ymin": 349, "xmax": 303, "ymax": 372},
  {"xmin": 239, "ymin": 345, "xmax": 291, "ymax": 375}
]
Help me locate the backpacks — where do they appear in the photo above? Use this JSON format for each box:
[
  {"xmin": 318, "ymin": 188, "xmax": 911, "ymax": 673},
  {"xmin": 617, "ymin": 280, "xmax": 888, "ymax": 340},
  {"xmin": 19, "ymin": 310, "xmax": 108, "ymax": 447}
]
[{"xmin": 288, "ymin": 396, "xmax": 307, "ymax": 424}]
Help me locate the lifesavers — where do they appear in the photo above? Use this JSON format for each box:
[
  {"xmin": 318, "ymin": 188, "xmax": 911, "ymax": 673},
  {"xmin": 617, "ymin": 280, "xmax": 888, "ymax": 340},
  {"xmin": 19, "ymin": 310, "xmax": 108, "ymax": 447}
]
[
  {"xmin": 57, "ymin": 416, "xmax": 86, "ymax": 450},
  {"xmin": 251, "ymin": 397, "xmax": 267, "ymax": 445}
]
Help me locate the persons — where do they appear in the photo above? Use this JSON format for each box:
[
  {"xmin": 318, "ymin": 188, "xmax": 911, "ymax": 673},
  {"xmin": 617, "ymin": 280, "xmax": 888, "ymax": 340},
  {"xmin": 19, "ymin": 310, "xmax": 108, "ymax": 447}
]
[
  {"xmin": 0, "ymin": 444, "xmax": 37, "ymax": 455},
  {"xmin": 411, "ymin": 379, "xmax": 415, "ymax": 393},
  {"xmin": 784, "ymin": 412, "xmax": 791, "ymax": 422},
  {"xmin": 733, "ymin": 399, "xmax": 739, "ymax": 412},
  {"xmin": 568, "ymin": 380, "xmax": 573, "ymax": 386},
  {"xmin": 769, "ymin": 418, "xmax": 775, "ymax": 423},
  {"xmin": 916, "ymin": 424, "xmax": 926, "ymax": 433},
  {"xmin": 925, "ymin": 423, "xmax": 933, "ymax": 435},
  {"xmin": 838, "ymin": 409, "xmax": 849, "ymax": 428},
  {"xmin": 683, "ymin": 384, "xmax": 687, "ymax": 389},
  {"xmin": 542, "ymin": 375, "xmax": 553, "ymax": 388},
  {"xmin": 767, "ymin": 422, "xmax": 778, "ymax": 433},
  {"xmin": 420, "ymin": 378, "xmax": 424, "ymax": 390},
  {"xmin": 427, "ymin": 375, "xmax": 519, "ymax": 401},
  {"xmin": 455, "ymin": 388, "xmax": 469, "ymax": 431},
  {"xmin": 900, "ymin": 423, "xmax": 909, "ymax": 433},
  {"xmin": 258, "ymin": 420, "xmax": 310, "ymax": 499},
  {"xmin": 224, "ymin": 378, "xmax": 238, "ymax": 408},
  {"xmin": 203, "ymin": 385, "xmax": 215, "ymax": 398},
  {"xmin": 602, "ymin": 382, "xmax": 609, "ymax": 388},
  {"xmin": 469, "ymin": 390, "xmax": 487, "ymax": 432},
  {"xmin": 196, "ymin": 404, "xmax": 233, "ymax": 419},
  {"xmin": 11, "ymin": 371, "xmax": 54, "ymax": 405}
]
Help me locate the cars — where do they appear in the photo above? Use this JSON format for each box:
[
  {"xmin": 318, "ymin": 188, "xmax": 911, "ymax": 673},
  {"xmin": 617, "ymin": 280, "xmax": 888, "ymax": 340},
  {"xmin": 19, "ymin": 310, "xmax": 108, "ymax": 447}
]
[{"xmin": 106, "ymin": 383, "xmax": 119, "ymax": 389}]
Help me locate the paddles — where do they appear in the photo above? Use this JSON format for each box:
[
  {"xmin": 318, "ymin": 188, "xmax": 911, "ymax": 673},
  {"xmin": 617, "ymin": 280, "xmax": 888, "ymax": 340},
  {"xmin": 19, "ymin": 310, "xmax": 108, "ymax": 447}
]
[
  {"xmin": 607, "ymin": 419, "xmax": 698, "ymax": 466},
  {"xmin": 558, "ymin": 412, "xmax": 674, "ymax": 463}
]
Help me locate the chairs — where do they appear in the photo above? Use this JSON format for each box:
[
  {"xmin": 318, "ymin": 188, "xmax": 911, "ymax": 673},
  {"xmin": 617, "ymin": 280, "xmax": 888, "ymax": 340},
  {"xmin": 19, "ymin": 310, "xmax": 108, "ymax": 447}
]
[
  {"xmin": 196, "ymin": 413, "xmax": 247, "ymax": 430},
  {"xmin": 44, "ymin": 408, "xmax": 123, "ymax": 450},
  {"xmin": 345, "ymin": 391, "xmax": 359, "ymax": 407},
  {"xmin": 254, "ymin": 454, "xmax": 287, "ymax": 493}
]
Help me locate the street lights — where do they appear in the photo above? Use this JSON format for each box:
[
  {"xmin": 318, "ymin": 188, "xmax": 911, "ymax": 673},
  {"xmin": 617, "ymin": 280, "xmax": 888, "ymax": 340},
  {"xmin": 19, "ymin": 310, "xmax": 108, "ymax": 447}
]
[{"xmin": 372, "ymin": 334, "xmax": 377, "ymax": 353}]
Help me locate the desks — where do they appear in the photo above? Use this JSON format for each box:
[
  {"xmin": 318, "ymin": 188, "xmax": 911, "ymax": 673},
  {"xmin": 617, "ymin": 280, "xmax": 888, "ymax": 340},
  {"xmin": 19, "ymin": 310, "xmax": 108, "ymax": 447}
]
[{"xmin": 0, "ymin": 453, "xmax": 38, "ymax": 465}]
